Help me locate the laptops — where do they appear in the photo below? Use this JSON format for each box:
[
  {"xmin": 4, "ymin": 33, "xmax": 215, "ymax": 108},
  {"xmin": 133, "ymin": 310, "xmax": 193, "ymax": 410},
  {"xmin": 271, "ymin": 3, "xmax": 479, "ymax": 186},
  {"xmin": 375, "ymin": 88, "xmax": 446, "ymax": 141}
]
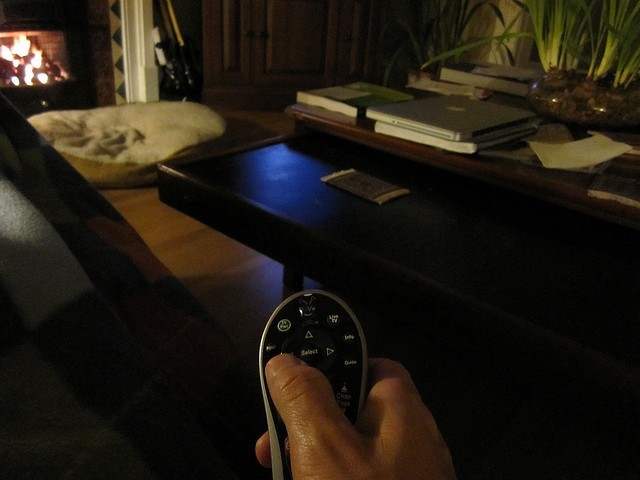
[{"xmin": 366, "ymin": 94, "xmax": 535, "ymax": 141}]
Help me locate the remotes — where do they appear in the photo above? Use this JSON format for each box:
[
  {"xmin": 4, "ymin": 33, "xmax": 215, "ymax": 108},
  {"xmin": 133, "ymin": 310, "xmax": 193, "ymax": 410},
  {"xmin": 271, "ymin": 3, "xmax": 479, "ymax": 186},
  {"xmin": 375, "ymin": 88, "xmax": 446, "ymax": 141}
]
[{"xmin": 257, "ymin": 288, "xmax": 367, "ymax": 480}]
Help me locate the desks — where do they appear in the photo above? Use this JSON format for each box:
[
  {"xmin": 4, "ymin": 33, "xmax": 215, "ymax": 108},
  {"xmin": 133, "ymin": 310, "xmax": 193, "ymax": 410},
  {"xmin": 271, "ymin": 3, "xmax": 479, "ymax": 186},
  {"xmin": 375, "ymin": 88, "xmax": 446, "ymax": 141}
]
[{"xmin": 155, "ymin": 89, "xmax": 639, "ymax": 422}]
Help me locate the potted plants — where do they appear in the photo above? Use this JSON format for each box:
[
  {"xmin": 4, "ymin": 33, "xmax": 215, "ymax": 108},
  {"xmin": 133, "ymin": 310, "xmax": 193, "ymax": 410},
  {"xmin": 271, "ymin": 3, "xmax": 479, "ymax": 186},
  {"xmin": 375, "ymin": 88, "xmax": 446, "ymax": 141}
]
[{"xmin": 420, "ymin": 0, "xmax": 639, "ymax": 135}]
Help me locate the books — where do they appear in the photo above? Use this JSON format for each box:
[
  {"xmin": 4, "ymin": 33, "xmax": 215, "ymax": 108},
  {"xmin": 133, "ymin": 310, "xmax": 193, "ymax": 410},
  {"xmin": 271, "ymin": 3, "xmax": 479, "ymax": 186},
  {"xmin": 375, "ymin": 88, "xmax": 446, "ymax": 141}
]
[
  {"xmin": 291, "ymin": 80, "xmax": 415, "ymax": 126},
  {"xmin": 365, "ymin": 95, "xmax": 538, "ymax": 154},
  {"xmin": 587, "ymin": 152, "xmax": 640, "ymax": 204}
]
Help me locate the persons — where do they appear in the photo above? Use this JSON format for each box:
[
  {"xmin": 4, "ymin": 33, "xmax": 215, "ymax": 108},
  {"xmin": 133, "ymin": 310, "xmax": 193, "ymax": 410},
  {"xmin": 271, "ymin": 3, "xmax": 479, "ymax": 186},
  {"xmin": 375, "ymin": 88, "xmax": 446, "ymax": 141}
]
[{"xmin": 255, "ymin": 352, "xmax": 457, "ymax": 478}]
